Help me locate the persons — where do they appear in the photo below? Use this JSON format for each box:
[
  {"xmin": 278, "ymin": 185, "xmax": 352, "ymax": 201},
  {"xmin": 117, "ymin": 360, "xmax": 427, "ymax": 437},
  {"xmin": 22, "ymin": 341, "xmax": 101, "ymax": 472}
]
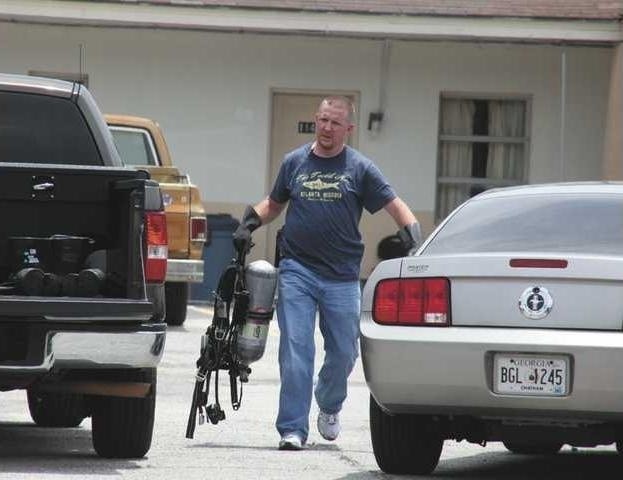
[{"xmin": 228, "ymin": 94, "xmax": 423, "ymax": 452}]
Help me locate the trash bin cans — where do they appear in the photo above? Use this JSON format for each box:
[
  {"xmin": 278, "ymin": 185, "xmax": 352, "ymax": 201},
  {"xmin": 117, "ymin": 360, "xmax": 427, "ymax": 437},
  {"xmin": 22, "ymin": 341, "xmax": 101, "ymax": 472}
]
[{"xmin": 186, "ymin": 209, "xmax": 243, "ymax": 308}]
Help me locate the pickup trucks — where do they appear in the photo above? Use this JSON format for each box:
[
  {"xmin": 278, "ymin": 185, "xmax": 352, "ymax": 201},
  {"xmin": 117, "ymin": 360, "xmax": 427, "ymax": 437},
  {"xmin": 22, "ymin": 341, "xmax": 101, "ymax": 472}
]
[
  {"xmin": 98, "ymin": 114, "xmax": 210, "ymax": 328},
  {"xmin": 0, "ymin": 70, "xmax": 169, "ymax": 460}
]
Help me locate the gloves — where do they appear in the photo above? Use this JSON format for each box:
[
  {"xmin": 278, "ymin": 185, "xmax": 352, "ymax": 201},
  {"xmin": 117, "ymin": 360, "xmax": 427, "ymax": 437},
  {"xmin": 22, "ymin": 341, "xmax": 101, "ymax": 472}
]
[
  {"xmin": 232, "ymin": 205, "xmax": 262, "ymax": 254},
  {"xmin": 396, "ymin": 220, "xmax": 422, "ymax": 261}
]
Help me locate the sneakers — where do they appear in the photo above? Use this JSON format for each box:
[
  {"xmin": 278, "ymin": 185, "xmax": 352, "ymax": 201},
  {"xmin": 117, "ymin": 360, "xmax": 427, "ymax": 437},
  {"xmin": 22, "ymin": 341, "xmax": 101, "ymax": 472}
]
[
  {"xmin": 278, "ymin": 433, "xmax": 308, "ymax": 450},
  {"xmin": 317, "ymin": 410, "xmax": 341, "ymax": 441}
]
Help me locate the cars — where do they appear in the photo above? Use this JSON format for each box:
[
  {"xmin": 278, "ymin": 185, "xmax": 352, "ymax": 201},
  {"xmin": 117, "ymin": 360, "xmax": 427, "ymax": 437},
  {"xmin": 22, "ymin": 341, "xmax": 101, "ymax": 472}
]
[{"xmin": 358, "ymin": 180, "xmax": 623, "ymax": 475}]
